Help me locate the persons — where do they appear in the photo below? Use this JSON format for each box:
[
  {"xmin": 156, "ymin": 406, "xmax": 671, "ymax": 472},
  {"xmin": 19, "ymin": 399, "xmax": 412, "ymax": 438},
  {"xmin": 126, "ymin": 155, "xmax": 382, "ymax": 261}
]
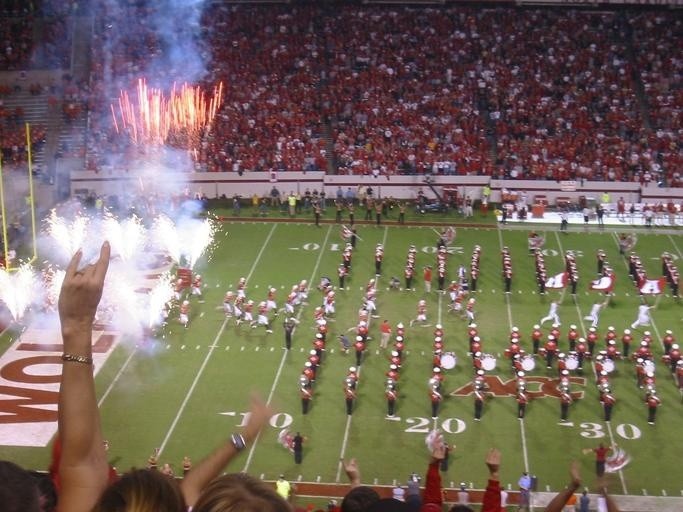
[{"xmin": 0, "ymin": 0, "xmax": 683, "ymax": 512}]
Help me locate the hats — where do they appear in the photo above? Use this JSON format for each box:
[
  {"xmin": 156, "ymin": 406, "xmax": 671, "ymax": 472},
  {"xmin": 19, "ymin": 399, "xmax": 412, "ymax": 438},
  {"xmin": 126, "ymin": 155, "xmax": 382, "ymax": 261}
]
[
  {"xmin": 470, "ymin": 323, "xmax": 484, "ymax": 376},
  {"xmin": 388, "ymin": 322, "xmax": 403, "ymax": 390},
  {"xmin": 503, "ymin": 247, "xmax": 679, "ymax": 281},
  {"xmin": 434, "ymin": 323, "xmax": 443, "ymax": 373},
  {"xmin": 511, "ymin": 326, "xmax": 525, "ymax": 378},
  {"xmin": 225, "ymin": 278, "xmax": 306, "ymax": 306},
  {"xmin": 356, "ymin": 335, "xmax": 362, "ymax": 343},
  {"xmin": 301, "ymin": 323, "xmax": 326, "ymax": 384},
  {"xmin": 349, "ymin": 367, "xmax": 356, "ymax": 373},
  {"xmin": 359, "ymin": 321, "xmax": 366, "ymax": 327},
  {"xmin": 338, "ymin": 242, "xmax": 480, "ymax": 277},
  {"xmin": 532, "ymin": 323, "xmax": 682, "ymax": 394}
]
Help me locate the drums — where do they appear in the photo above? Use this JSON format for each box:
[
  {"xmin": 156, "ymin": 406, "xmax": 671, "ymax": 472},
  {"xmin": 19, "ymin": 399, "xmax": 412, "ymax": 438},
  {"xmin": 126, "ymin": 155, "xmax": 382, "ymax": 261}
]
[
  {"xmin": 642, "ymin": 361, "xmax": 655, "ymax": 374},
  {"xmin": 602, "ymin": 359, "xmax": 615, "ymax": 373},
  {"xmin": 565, "ymin": 356, "xmax": 578, "ymax": 370},
  {"xmin": 440, "ymin": 352, "xmax": 457, "ymax": 370},
  {"xmin": 481, "ymin": 354, "xmax": 496, "ymax": 371},
  {"xmin": 521, "ymin": 356, "xmax": 535, "ymax": 371}
]
